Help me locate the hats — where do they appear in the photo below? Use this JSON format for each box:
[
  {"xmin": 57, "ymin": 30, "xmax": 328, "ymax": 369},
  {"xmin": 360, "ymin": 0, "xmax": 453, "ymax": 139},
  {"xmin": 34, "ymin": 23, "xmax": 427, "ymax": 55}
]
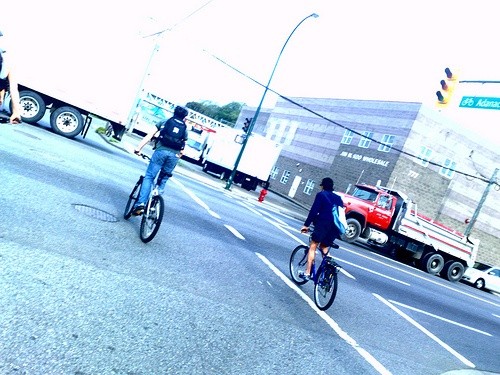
[{"xmin": 320, "ymin": 178, "xmax": 333, "ymax": 186}]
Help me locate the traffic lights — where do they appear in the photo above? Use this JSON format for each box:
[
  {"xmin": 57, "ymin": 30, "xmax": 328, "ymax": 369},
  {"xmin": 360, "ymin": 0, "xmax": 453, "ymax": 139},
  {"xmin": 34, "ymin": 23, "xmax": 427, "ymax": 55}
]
[
  {"xmin": 242, "ymin": 117, "xmax": 254, "ymax": 135},
  {"xmin": 436, "ymin": 67, "xmax": 460, "ymax": 107}
]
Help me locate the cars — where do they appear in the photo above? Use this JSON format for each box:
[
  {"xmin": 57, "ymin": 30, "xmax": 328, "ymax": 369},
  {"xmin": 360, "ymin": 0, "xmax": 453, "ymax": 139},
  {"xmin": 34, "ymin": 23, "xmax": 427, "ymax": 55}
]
[{"xmin": 461, "ymin": 260, "xmax": 500, "ymax": 294}]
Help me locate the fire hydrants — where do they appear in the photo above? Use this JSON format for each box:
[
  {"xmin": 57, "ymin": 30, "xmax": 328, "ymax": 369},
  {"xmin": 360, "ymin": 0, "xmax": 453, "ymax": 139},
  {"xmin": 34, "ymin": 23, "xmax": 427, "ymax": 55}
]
[{"xmin": 258, "ymin": 189, "xmax": 267, "ymax": 203}]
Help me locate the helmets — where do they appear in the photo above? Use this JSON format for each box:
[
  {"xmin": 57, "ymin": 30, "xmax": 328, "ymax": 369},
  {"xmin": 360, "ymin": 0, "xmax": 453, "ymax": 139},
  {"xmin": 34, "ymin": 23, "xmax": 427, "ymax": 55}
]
[{"xmin": 174, "ymin": 106, "xmax": 188, "ymax": 116}]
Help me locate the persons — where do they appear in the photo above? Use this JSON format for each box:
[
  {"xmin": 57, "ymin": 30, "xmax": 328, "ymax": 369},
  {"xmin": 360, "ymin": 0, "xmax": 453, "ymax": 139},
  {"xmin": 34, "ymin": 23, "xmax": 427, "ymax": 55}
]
[
  {"xmin": 0, "ymin": 29, "xmax": 23, "ymax": 125},
  {"xmin": 298, "ymin": 170, "xmax": 346, "ymax": 280},
  {"xmin": 132, "ymin": 103, "xmax": 186, "ymax": 217}
]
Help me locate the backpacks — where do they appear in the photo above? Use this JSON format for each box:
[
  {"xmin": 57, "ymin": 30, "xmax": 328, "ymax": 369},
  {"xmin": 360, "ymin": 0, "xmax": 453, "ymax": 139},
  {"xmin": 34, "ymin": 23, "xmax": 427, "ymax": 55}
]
[{"xmin": 160, "ymin": 116, "xmax": 186, "ymax": 150}]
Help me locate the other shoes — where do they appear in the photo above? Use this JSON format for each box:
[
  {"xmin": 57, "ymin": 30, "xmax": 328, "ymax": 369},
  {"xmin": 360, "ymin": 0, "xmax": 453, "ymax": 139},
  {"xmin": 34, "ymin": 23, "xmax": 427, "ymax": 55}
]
[
  {"xmin": 299, "ymin": 273, "xmax": 310, "ymax": 281},
  {"xmin": 132, "ymin": 205, "xmax": 144, "ymax": 213}
]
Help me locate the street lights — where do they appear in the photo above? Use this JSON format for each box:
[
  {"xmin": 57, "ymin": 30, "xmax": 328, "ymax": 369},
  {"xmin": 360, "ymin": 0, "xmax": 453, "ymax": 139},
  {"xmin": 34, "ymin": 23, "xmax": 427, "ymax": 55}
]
[{"xmin": 225, "ymin": 12, "xmax": 319, "ymax": 191}]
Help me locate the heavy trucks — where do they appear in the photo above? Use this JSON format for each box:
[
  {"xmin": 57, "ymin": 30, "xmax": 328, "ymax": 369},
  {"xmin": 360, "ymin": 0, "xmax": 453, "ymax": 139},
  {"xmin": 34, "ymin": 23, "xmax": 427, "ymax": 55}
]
[
  {"xmin": 332, "ymin": 180, "xmax": 481, "ymax": 283},
  {"xmin": 0, "ymin": 0, "xmax": 158, "ymax": 139}
]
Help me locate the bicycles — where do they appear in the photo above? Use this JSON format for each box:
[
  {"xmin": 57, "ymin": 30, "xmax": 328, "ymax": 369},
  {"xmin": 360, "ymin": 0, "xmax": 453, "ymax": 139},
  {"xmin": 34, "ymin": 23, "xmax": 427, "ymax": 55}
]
[
  {"xmin": 289, "ymin": 228, "xmax": 342, "ymax": 311},
  {"xmin": 124, "ymin": 151, "xmax": 173, "ymax": 244}
]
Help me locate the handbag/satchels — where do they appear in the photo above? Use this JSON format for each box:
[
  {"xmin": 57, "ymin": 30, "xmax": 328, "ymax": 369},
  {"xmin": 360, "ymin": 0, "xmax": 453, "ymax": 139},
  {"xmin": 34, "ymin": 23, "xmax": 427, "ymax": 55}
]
[{"xmin": 332, "ymin": 205, "xmax": 348, "ymax": 235}]
